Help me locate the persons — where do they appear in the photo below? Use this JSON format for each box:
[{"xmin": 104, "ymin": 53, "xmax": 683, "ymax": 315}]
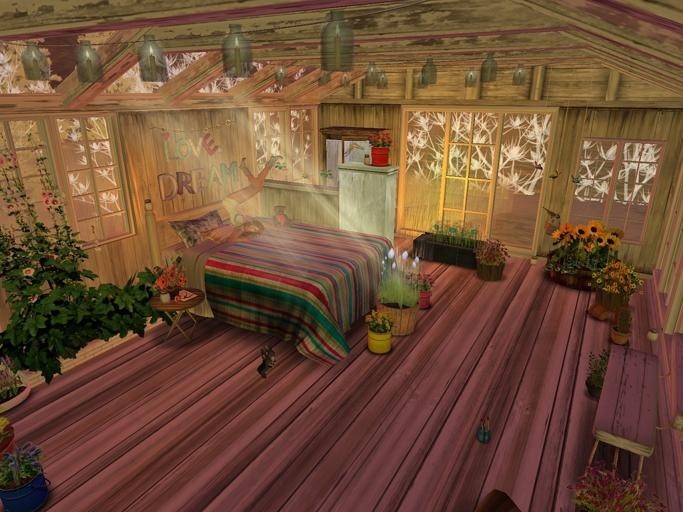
[{"xmin": 223, "ymin": 154, "xmax": 282, "ymax": 243}]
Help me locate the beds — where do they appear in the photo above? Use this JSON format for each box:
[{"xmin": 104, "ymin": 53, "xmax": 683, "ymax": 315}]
[{"xmin": 143, "ymin": 198, "xmax": 396, "ymax": 346}]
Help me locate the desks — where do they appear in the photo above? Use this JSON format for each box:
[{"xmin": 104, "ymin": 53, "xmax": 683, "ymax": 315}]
[{"xmin": 148, "ymin": 288, "xmax": 205, "ymax": 344}]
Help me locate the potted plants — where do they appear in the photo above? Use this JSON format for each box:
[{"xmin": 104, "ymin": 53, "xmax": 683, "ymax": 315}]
[
  {"xmin": 585, "ymin": 306, "xmax": 659, "ymax": 400},
  {"xmin": 412, "ymin": 223, "xmax": 479, "ymax": 269}
]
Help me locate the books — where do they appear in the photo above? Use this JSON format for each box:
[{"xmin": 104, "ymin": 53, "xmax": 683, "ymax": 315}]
[{"xmin": 174, "ymin": 288, "xmax": 197, "ymax": 302}]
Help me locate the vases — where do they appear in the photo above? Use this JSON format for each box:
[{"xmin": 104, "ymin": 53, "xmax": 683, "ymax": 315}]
[
  {"xmin": 0, "ymin": 471, "xmax": 47, "ymax": 511},
  {"xmin": 366, "ymin": 329, "xmax": 392, "ymax": 354},
  {"xmin": 475, "ymin": 263, "xmax": 505, "ymax": 281},
  {"xmin": 370, "ymin": 147, "xmax": 389, "ymax": 165},
  {"xmin": 158, "ymin": 293, "xmax": 170, "ymax": 304}
]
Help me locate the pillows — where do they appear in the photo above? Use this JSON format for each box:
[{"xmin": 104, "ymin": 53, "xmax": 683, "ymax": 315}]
[{"xmin": 167, "ymin": 209, "xmax": 223, "ymax": 248}]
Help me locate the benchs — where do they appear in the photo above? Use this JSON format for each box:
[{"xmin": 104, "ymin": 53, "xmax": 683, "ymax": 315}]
[{"xmin": 576, "ymin": 344, "xmax": 659, "ymax": 482}]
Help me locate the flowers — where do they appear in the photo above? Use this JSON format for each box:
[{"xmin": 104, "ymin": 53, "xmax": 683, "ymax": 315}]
[
  {"xmin": 541, "ymin": 219, "xmax": 650, "ymax": 311},
  {"xmin": 362, "ymin": 247, "xmax": 434, "ymax": 334},
  {"xmin": 0, "ymin": 343, "xmax": 43, "ymax": 487},
  {"xmin": 473, "ymin": 238, "xmax": 512, "ymax": 267},
  {"xmin": 150, "ymin": 255, "xmax": 187, "ymax": 294},
  {"xmin": 367, "ymin": 130, "xmax": 393, "ymax": 147}
]
